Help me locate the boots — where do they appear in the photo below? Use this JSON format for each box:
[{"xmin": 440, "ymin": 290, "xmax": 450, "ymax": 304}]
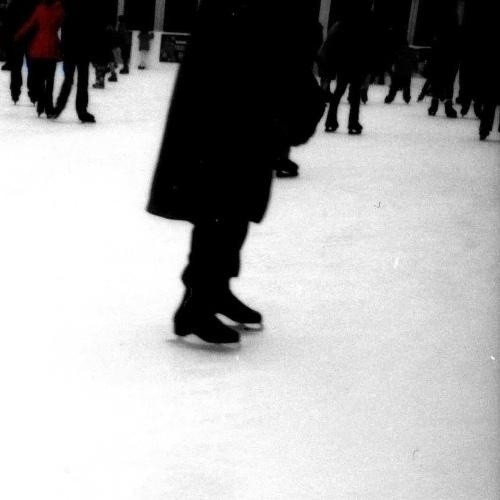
[
  {"xmin": 173, "ymin": 277, "xmax": 240, "ymax": 344},
  {"xmin": 384, "ymin": 84, "xmax": 500, "ymax": 138},
  {"xmin": 4, "ymin": 86, "xmax": 97, "ymax": 120},
  {"xmin": 89, "ymin": 65, "xmax": 146, "ymax": 88},
  {"xmin": 278, "ymin": 157, "xmax": 300, "ymax": 171},
  {"xmin": 322, "ymin": 88, "xmax": 369, "ymax": 129},
  {"xmin": 202, "ymin": 277, "xmax": 264, "ymax": 326}
]
